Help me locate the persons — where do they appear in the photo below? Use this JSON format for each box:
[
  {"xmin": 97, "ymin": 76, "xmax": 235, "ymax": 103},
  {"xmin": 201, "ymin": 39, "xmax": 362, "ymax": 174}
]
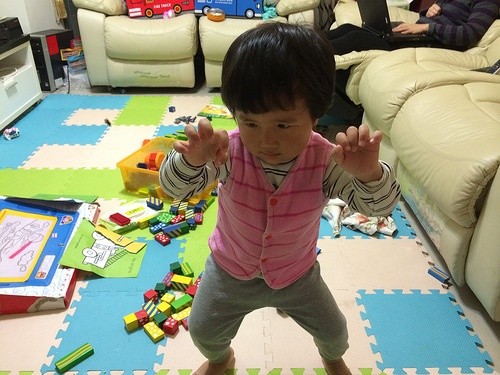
[
  {"xmin": 159, "ymin": 23, "xmax": 402, "ymax": 375},
  {"xmin": 323, "ymin": 0, "xmax": 500, "ymax": 54}
]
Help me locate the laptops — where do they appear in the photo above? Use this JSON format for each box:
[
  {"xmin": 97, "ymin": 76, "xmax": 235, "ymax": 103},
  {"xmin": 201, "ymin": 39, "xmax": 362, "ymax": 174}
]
[{"xmin": 357, "ymin": 0, "xmax": 437, "ymax": 43}]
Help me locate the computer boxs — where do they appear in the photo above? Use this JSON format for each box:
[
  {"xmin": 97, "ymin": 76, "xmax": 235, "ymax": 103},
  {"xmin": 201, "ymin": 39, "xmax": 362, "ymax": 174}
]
[{"xmin": 27, "ymin": 29, "xmax": 73, "ymax": 92}]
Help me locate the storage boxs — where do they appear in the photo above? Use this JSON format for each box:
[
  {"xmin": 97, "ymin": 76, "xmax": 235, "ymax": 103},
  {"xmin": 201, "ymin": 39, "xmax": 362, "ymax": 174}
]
[{"xmin": 116, "ymin": 137, "xmax": 218, "ymax": 206}]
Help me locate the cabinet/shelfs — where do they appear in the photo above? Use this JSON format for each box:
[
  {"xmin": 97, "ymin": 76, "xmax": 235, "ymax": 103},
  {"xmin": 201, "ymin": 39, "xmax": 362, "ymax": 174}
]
[{"xmin": 0, "ymin": 34, "xmax": 44, "ymax": 132}]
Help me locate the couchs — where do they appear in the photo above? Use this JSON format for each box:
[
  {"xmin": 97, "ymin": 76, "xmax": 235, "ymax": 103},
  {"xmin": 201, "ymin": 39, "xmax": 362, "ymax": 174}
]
[{"xmin": 73, "ymin": 0, "xmax": 500, "ymax": 323}]
